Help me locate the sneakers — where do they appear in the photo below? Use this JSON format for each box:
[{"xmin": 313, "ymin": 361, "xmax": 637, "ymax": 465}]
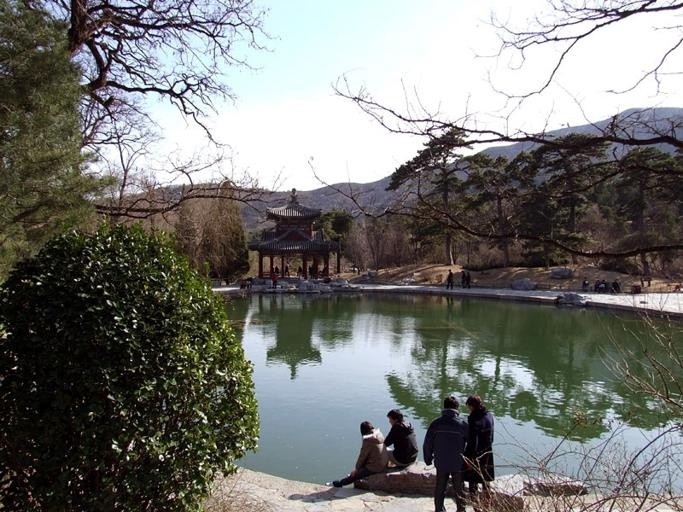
[{"xmin": 325, "ymin": 482, "xmax": 333, "ymax": 486}]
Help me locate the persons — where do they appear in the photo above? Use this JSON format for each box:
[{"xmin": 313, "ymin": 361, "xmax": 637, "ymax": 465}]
[
  {"xmin": 239, "ymin": 274, "xmax": 252, "ymax": 288},
  {"xmin": 446, "ymin": 269, "xmax": 453, "ymax": 289},
  {"xmin": 583, "ymin": 277, "xmax": 624, "ymax": 295},
  {"xmin": 640, "ymin": 273, "xmax": 652, "ymax": 287},
  {"xmin": 297, "ymin": 262, "xmax": 329, "ymax": 278},
  {"xmin": 384, "ymin": 409, "xmax": 418, "ymax": 471},
  {"xmin": 270, "ymin": 264, "xmax": 289, "ymax": 289},
  {"xmin": 465, "ymin": 395, "xmax": 494, "ymax": 512},
  {"xmin": 325, "ymin": 420, "xmax": 390, "ymax": 488},
  {"xmin": 422, "ymin": 396, "xmax": 471, "ymax": 512},
  {"xmin": 461, "ymin": 271, "xmax": 471, "ymax": 288}
]
